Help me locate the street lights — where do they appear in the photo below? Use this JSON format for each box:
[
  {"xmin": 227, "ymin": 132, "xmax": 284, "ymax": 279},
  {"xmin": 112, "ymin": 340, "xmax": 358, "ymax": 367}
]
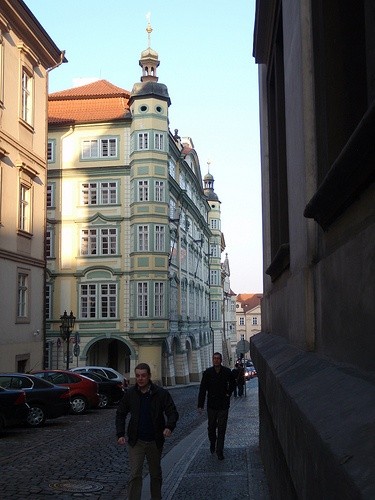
[{"xmin": 59, "ymin": 310, "xmax": 76, "ymax": 370}]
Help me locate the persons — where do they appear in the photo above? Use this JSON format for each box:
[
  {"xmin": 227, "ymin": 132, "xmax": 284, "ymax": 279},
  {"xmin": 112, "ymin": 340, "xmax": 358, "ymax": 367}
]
[
  {"xmin": 196, "ymin": 353, "xmax": 238, "ymax": 460},
  {"xmin": 230, "ymin": 362, "xmax": 247, "ymax": 399},
  {"xmin": 113, "ymin": 362, "xmax": 180, "ymax": 500}
]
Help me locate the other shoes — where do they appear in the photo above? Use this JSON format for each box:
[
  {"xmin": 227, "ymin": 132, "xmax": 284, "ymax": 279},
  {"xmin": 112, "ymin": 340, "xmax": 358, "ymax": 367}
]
[
  {"xmin": 210, "ymin": 445, "xmax": 216, "ymax": 454},
  {"xmin": 216, "ymin": 451, "xmax": 224, "ymax": 459}
]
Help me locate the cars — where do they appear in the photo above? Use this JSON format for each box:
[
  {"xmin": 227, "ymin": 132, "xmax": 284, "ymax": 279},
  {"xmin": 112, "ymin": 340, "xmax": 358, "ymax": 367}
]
[
  {"xmin": 242, "ymin": 359, "xmax": 257, "ymax": 381},
  {"xmin": 0, "ymin": 360, "xmax": 129, "ymax": 438}
]
